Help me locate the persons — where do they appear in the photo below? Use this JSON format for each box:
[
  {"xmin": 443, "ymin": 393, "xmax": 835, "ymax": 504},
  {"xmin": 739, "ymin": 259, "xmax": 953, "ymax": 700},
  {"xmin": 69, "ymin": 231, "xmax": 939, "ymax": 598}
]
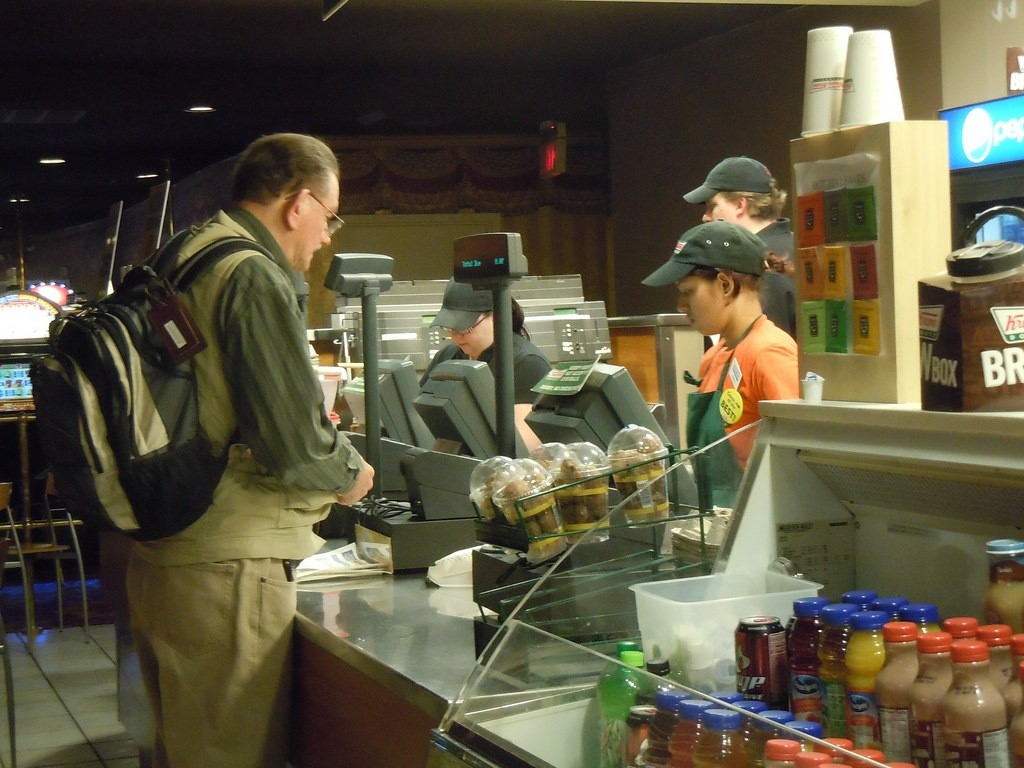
[
  {"xmin": 684, "ymin": 157, "xmax": 797, "ymax": 340},
  {"xmin": 641, "ymin": 219, "xmax": 798, "ymax": 513},
  {"xmin": 116, "ymin": 135, "xmax": 374, "ymax": 768},
  {"xmin": 418, "ymin": 276, "xmax": 553, "ymax": 454}
]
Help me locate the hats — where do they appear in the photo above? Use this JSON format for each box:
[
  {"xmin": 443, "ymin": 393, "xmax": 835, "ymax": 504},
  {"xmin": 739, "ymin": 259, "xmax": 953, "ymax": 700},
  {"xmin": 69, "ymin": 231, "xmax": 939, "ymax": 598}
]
[
  {"xmin": 641, "ymin": 218, "xmax": 768, "ymax": 287},
  {"xmin": 681, "ymin": 155, "xmax": 774, "ymax": 208},
  {"xmin": 429, "ymin": 276, "xmax": 494, "ymax": 331}
]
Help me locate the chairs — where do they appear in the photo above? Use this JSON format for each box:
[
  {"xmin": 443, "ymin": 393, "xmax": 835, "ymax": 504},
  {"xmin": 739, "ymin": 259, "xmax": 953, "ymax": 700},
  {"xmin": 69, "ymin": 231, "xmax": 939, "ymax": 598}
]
[{"xmin": 0, "ymin": 471, "xmax": 91, "ymax": 644}]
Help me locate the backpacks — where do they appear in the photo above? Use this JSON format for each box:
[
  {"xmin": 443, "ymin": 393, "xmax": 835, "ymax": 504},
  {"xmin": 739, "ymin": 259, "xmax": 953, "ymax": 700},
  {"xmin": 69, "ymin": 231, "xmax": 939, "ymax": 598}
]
[{"xmin": 29, "ymin": 226, "xmax": 277, "ymax": 542}]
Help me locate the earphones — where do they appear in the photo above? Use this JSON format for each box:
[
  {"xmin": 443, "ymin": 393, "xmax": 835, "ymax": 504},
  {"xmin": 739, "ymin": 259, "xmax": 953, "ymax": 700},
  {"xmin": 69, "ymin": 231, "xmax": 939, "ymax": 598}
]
[{"xmin": 722, "ymin": 280, "xmax": 730, "ymax": 290}]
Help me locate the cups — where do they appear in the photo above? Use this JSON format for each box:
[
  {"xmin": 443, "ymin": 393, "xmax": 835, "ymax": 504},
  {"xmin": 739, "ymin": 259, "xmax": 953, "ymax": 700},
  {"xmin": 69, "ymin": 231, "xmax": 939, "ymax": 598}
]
[
  {"xmin": 472, "ymin": 450, "xmax": 668, "ymax": 560},
  {"xmin": 801, "ymin": 380, "xmax": 823, "ymax": 401},
  {"xmin": 802, "ymin": 27, "xmax": 854, "ymax": 136},
  {"xmin": 836, "ymin": 30, "xmax": 905, "ymax": 129}
]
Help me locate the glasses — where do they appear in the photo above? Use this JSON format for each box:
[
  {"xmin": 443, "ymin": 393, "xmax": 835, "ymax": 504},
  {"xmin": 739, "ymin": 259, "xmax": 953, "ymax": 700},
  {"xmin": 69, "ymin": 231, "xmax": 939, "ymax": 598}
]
[
  {"xmin": 284, "ymin": 189, "xmax": 345, "ymax": 237},
  {"xmin": 439, "ymin": 315, "xmax": 490, "ymax": 335}
]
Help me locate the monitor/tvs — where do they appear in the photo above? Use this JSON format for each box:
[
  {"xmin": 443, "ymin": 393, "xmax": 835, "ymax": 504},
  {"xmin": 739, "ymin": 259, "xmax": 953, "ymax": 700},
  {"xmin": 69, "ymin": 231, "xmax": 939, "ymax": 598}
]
[
  {"xmin": 340, "ymin": 358, "xmax": 435, "ymax": 452},
  {"xmin": 412, "ymin": 361, "xmax": 529, "ymax": 460},
  {"xmin": 0, "ymin": 362, "xmax": 36, "ymax": 399},
  {"xmin": 524, "ymin": 363, "xmax": 698, "ymax": 516}
]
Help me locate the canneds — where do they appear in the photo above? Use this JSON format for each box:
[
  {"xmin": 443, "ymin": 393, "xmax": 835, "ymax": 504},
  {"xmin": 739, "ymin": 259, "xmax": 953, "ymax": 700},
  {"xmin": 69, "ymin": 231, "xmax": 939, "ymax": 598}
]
[
  {"xmin": 624, "ymin": 704, "xmax": 657, "ymax": 768},
  {"xmin": 734, "ymin": 615, "xmax": 789, "ymax": 714}
]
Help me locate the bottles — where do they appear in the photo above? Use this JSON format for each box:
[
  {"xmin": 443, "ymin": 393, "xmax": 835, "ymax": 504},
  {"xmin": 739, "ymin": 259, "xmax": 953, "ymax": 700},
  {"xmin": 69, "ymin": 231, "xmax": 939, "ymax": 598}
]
[{"xmin": 597, "ymin": 540, "xmax": 1024, "ymax": 768}]
